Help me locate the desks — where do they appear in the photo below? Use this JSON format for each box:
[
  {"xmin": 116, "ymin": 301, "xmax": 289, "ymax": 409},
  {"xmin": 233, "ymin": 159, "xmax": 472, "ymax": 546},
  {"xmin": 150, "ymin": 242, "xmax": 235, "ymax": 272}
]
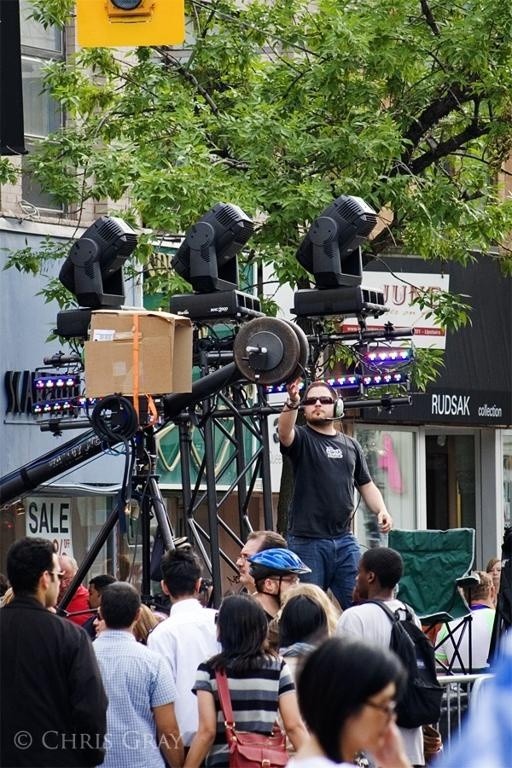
[{"xmin": 436, "ymin": 673, "xmax": 493, "ymax": 755}]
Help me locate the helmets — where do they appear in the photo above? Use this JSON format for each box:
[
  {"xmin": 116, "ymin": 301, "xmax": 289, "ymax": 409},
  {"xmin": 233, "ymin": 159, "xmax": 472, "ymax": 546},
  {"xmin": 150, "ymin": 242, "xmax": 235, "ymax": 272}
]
[{"xmin": 248, "ymin": 549, "xmax": 312, "ymax": 578}]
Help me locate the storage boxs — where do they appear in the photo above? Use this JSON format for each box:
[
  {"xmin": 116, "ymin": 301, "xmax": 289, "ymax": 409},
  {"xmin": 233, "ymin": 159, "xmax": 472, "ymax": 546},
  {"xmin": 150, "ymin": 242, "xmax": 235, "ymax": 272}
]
[{"xmin": 83, "ymin": 306, "xmax": 194, "ymax": 402}]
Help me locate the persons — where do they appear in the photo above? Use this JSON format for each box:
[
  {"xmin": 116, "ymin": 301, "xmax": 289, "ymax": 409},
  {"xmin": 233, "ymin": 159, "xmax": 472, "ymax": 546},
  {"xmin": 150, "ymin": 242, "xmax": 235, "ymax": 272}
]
[
  {"xmin": 0, "ymin": 530, "xmax": 512, "ymax": 768},
  {"xmin": 277, "ymin": 376, "xmax": 393, "ymax": 611}
]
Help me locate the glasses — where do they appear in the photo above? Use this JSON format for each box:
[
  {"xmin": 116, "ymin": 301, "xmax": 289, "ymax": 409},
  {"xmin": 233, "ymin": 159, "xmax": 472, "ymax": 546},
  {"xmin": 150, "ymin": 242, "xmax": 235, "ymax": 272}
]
[
  {"xmin": 96, "ymin": 612, "xmax": 103, "ymax": 621},
  {"xmin": 366, "ymin": 699, "xmax": 396, "ymax": 715},
  {"xmin": 49, "ymin": 569, "xmax": 66, "ymax": 578},
  {"xmin": 303, "ymin": 396, "xmax": 334, "ymax": 406}
]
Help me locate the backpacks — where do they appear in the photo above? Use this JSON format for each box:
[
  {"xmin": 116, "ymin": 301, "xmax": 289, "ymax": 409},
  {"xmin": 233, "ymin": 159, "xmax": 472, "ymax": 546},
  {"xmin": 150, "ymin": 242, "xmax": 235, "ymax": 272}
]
[{"xmin": 368, "ymin": 598, "xmax": 448, "ymax": 728}]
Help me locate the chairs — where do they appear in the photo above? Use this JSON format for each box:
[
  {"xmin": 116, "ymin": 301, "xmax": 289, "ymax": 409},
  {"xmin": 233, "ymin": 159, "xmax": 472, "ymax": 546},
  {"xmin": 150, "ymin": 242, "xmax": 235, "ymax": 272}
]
[{"xmin": 387, "ymin": 528, "xmax": 480, "ymax": 673}]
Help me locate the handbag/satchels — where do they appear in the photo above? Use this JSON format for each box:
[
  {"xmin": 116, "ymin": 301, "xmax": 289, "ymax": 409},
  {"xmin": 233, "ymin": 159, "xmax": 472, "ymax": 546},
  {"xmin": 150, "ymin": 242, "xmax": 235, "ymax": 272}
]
[
  {"xmin": 224, "ymin": 726, "xmax": 290, "ymax": 767},
  {"xmin": 422, "ymin": 725, "xmax": 443, "ymax": 766}
]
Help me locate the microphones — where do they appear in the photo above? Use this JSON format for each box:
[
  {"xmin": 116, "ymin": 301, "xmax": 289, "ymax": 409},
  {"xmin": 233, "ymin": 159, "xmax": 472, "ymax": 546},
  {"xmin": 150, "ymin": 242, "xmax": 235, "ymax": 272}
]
[{"xmin": 322, "ymin": 412, "xmax": 349, "ymax": 426}]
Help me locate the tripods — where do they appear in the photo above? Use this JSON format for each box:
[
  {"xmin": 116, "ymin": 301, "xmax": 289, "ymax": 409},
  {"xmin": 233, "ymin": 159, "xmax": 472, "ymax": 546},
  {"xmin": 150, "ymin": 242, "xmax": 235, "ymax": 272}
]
[{"xmin": 42, "ymin": 474, "xmax": 204, "ymax": 621}]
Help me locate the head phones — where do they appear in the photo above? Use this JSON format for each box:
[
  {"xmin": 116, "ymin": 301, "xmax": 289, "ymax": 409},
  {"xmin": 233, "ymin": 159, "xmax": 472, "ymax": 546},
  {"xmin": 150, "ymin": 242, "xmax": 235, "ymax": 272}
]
[{"xmin": 333, "ymin": 377, "xmax": 348, "ymax": 420}]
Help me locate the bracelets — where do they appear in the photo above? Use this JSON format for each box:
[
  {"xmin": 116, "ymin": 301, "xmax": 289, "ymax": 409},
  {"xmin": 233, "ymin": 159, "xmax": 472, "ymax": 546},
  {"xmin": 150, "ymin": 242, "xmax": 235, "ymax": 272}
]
[{"xmin": 286, "ymin": 397, "xmax": 300, "ymax": 410}]
